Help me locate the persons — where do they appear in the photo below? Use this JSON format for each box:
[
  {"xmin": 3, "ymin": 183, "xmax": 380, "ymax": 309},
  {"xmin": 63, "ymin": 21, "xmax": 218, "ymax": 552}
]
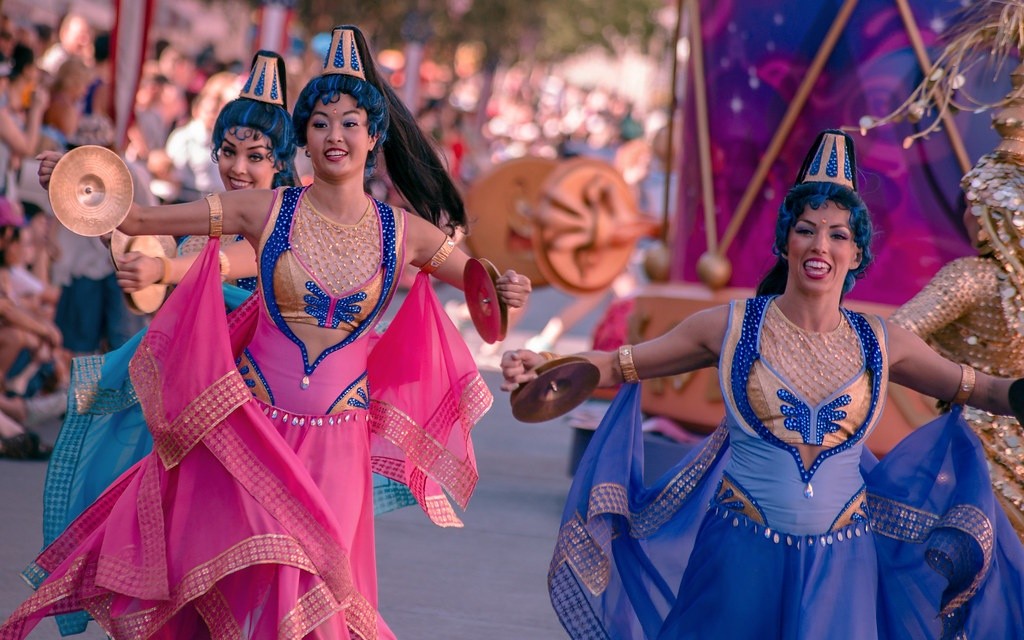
[
  {"xmin": 79, "ymin": 99, "xmax": 339, "ymax": 640},
  {"xmin": 35, "ymin": 76, "xmax": 532, "ymax": 640},
  {"xmin": 0, "ymin": 1, "xmax": 1022, "ymax": 639},
  {"xmin": 498, "ymin": 182, "xmax": 1024, "ymax": 637}
]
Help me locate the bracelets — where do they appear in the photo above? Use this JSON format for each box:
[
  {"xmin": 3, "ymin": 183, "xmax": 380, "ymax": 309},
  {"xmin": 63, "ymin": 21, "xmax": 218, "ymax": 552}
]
[{"xmin": 155, "ymin": 256, "xmax": 171, "ymax": 285}]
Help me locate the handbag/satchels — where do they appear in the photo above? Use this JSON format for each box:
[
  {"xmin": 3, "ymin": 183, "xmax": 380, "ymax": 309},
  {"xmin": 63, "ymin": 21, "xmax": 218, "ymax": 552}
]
[{"xmin": 0, "ymin": 170, "xmax": 25, "ymax": 228}]
